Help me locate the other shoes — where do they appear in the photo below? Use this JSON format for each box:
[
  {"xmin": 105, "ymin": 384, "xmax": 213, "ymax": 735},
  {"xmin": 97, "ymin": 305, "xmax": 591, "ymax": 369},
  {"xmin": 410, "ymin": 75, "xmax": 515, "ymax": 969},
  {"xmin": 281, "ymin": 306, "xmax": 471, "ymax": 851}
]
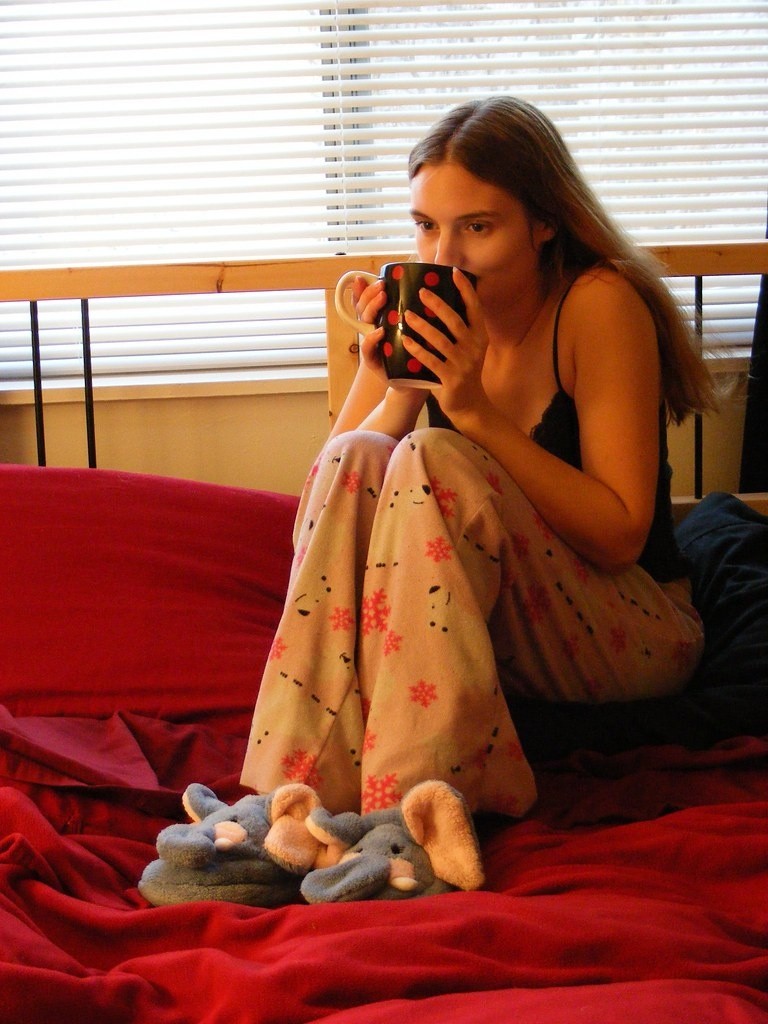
[
  {"xmin": 138, "ymin": 782, "xmax": 322, "ymax": 908},
  {"xmin": 300, "ymin": 779, "xmax": 487, "ymax": 903}
]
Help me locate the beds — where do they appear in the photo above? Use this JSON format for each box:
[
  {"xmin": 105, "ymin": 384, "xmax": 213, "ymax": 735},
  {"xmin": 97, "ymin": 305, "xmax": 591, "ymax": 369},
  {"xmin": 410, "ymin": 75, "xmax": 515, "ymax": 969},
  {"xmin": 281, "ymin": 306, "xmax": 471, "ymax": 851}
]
[{"xmin": 0, "ymin": 242, "xmax": 768, "ymax": 1024}]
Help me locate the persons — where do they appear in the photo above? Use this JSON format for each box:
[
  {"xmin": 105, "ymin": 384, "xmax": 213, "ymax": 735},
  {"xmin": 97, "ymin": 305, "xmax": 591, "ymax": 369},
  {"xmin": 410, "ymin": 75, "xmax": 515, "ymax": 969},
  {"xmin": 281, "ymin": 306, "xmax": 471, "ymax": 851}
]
[{"xmin": 137, "ymin": 97, "xmax": 720, "ymax": 907}]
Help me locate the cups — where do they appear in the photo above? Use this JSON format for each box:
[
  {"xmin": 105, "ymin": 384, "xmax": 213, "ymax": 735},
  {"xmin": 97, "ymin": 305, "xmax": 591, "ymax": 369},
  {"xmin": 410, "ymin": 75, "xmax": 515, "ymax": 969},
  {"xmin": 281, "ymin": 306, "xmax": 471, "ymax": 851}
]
[{"xmin": 335, "ymin": 263, "xmax": 478, "ymax": 390}]
